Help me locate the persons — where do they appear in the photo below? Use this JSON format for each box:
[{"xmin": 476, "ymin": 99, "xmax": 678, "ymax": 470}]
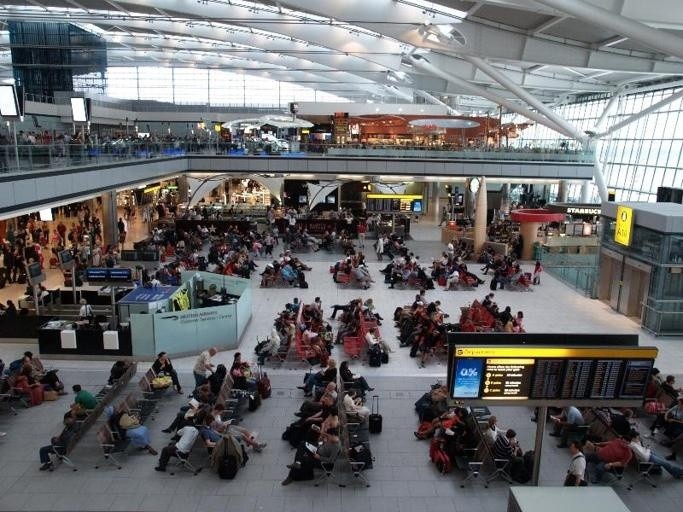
[
  {"xmin": 0, "ymin": 124, "xmax": 239, "ymax": 180},
  {"xmin": 549, "ymin": 365, "xmax": 682, "ymax": 489},
  {"xmin": 1, "ymin": 180, "xmax": 550, "ymax": 484}
]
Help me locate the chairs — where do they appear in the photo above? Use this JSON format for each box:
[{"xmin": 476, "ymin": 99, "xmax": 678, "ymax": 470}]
[{"xmin": 256, "ymin": 299, "xmax": 527, "ymax": 369}]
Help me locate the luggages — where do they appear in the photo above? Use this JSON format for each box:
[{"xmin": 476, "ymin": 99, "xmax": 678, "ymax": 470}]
[
  {"xmin": 369, "ymin": 396, "xmax": 382, "ymax": 433},
  {"xmin": 258, "ymin": 364, "xmax": 271, "ymax": 398},
  {"xmin": 369, "ymin": 338, "xmax": 381, "ymax": 367},
  {"xmin": 219, "ymin": 437, "xmax": 237, "ymax": 480},
  {"xmin": 435, "ymin": 449, "xmax": 451, "ymax": 475}
]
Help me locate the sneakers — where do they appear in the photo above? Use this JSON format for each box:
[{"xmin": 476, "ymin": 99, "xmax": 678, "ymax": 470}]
[
  {"xmin": 369, "ymin": 388, "xmax": 374, "ymax": 391},
  {"xmin": 550, "ymin": 430, "xmax": 567, "ymax": 449},
  {"xmin": 394, "ymin": 320, "xmax": 408, "ymax": 347},
  {"xmin": 297, "ymin": 385, "xmax": 305, "ymax": 389},
  {"xmin": 287, "ymin": 463, "xmax": 302, "ymax": 470},
  {"xmin": 148, "ymin": 447, "xmax": 158, "ymax": 455},
  {"xmin": 253, "ymin": 439, "xmax": 267, "ymax": 452},
  {"xmin": 295, "ymin": 412, "xmax": 305, "ymax": 417},
  {"xmin": 161, "ymin": 427, "xmax": 172, "ymax": 433},
  {"xmin": 414, "ymin": 431, "xmax": 428, "ymax": 441},
  {"xmin": 155, "ymin": 466, "xmax": 166, "ymax": 471},
  {"xmin": 39, "ymin": 462, "xmax": 53, "ymax": 470},
  {"xmin": 282, "ymin": 478, "xmax": 293, "ymax": 485}
]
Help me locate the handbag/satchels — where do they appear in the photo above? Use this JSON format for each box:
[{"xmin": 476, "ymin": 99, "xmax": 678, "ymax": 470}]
[
  {"xmin": 249, "ymin": 392, "xmax": 261, "ymax": 411},
  {"xmin": 120, "ymin": 413, "xmax": 139, "ymax": 427},
  {"xmin": 382, "ymin": 353, "xmax": 389, "ymax": 364},
  {"xmin": 44, "ymin": 390, "xmax": 58, "ymax": 401},
  {"xmin": 350, "ymin": 443, "xmax": 373, "ymax": 471},
  {"xmin": 152, "ymin": 376, "xmax": 173, "ymax": 388}
]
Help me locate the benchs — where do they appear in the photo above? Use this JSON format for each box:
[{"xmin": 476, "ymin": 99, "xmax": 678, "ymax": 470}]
[
  {"xmin": 309, "ymin": 379, "xmax": 373, "ymax": 487},
  {"xmin": 50, "ymin": 360, "xmax": 138, "ymax": 472},
  {"xmin": 448, "ymin": 402, "xmax": 512, "ymax": 488},
  {"xmin": 170, "ymin": 365, "xmax": 247, "ymax": 476},
  {"xmin": 93, "ymin": 358, "xmax": 169, "ymax": 469},
  {"xmin": 582, "ymin": 380, "xmax": 682, "ymax": 490}
]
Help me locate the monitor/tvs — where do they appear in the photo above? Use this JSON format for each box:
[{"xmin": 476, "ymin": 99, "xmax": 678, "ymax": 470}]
[
  {"xmin": 25, "ymin": 263, "xmax": 46, "ymax": 285},
  {"xmin": 142, "ymin": 249, "xmax": 159, "ymax": 261},
  {"xmin": 121, "ymin": 250, "xmax": 138, "ymax": 261},
  {"xmin": 108, "ymin": 269, "xmax": 132, "ymax": 282},
  {"xmin": 86, "ymin": 268, "xmax": 108, "ymax": 282},
  {"xmin": 58, "ymin": 249, "xmax": 75, "ymax": 271}
]
